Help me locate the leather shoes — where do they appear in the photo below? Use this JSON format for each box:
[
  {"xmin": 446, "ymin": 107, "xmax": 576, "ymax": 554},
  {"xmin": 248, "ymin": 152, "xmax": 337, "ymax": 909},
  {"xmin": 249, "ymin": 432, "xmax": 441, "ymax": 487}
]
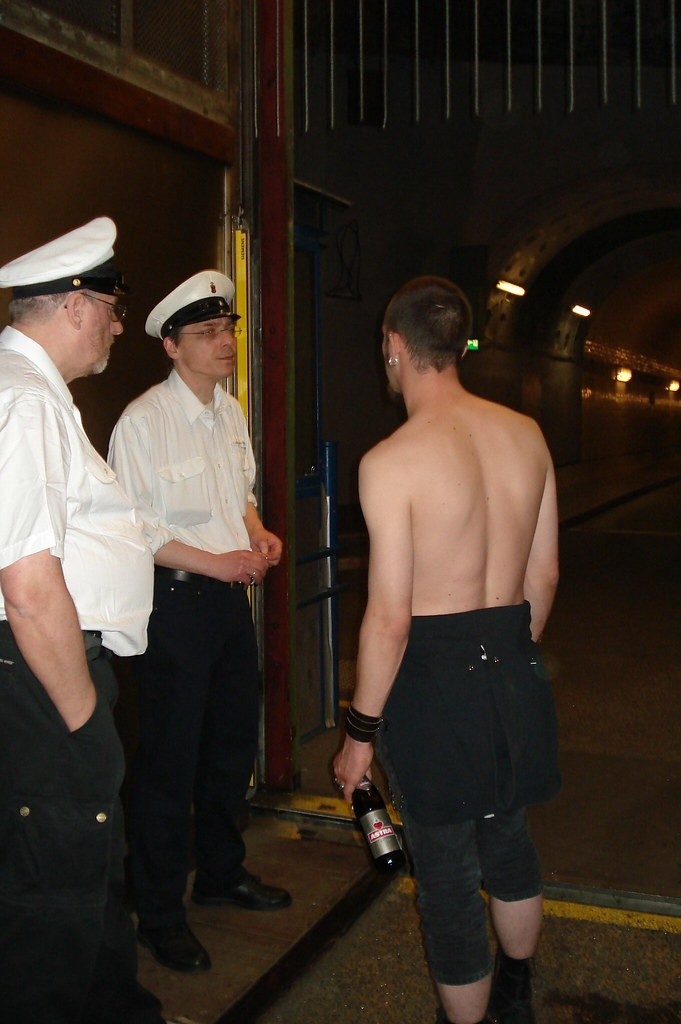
[
  {"xmin": 137, "ymin": 923, "xmax": 209, "ymax": 975},
  {"xmin": 193, "ymin": 873, "xmax": 293, "ymax": 910}
]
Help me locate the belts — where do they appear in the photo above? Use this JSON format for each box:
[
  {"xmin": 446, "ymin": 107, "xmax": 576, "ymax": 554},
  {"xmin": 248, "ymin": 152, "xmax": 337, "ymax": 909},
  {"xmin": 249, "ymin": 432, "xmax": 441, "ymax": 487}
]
[
  {"xmin": 82, "ymin": 630, "xmax": 102, "ymax": 640},
  {"xmin": 154, "ymin": 566, "xmax": 217, "ymax": 584}
]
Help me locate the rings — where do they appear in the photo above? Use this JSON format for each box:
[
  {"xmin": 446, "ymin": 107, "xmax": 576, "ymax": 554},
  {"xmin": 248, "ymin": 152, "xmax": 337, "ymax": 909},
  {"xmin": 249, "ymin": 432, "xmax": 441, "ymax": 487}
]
[
  {"xmin": 338, "ymin": 784, "xmax": 344, "ymax": 791},
  {"xmin": 333, "ymin": 777, "xmax": 338, "ymax": 783},
  {"xmin": 252, "ymin": 571, "xmax": 256, "ymax": 575}
]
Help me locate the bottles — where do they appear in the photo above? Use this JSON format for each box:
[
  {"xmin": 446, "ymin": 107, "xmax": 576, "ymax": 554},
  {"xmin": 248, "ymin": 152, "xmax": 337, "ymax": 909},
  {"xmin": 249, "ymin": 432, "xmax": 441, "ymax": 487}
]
[{"xmin": 347, "ymin": 775, "xmax": 408, "ymax": 876}]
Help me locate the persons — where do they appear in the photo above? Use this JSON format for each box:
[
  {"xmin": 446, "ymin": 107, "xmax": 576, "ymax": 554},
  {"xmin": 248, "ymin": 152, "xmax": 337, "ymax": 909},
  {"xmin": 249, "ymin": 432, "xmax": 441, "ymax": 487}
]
[
  {"xmin": 0, "ymin": 213, "xmax": 280, "ymax": 1024},
  {"xmin": 331, "ymin": 274, "xmax": 563, "ymax": 1024}
]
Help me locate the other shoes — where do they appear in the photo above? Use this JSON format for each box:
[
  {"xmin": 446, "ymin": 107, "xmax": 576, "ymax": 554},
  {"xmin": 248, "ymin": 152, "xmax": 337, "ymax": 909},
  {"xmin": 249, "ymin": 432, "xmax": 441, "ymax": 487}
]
[{"xmin": 487, "ymin": 996, "xmax": 536, "ymax": 1024}]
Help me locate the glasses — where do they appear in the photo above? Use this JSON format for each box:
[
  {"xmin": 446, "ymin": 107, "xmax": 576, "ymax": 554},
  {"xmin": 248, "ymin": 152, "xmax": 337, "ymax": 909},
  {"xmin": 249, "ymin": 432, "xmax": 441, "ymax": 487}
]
[
  {"xmin": 171, "ymin": 325, "xmax": 242, "ymax": 343},
  {"xmin": 64, "ymin": 288, "xmax": 126, "ymax": 324}
]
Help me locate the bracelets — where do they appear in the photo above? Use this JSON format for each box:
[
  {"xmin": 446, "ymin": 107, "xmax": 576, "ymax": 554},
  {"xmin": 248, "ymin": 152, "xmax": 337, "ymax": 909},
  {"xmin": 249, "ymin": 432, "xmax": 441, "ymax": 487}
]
[{"xmin": 344, "ymin": 701, "xmax": 384, "ymax": 742}]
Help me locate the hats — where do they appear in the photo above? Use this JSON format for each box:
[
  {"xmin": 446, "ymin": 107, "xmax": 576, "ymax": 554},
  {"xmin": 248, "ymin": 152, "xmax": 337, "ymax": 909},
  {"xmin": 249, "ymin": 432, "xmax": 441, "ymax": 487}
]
[
  {"xmin": 0, "ymin": 216, "xmax": 138, "ymax": 303},
  {"xmin": 145, "ymin": 272, "xmax": 242, "ymax": 341}
]
